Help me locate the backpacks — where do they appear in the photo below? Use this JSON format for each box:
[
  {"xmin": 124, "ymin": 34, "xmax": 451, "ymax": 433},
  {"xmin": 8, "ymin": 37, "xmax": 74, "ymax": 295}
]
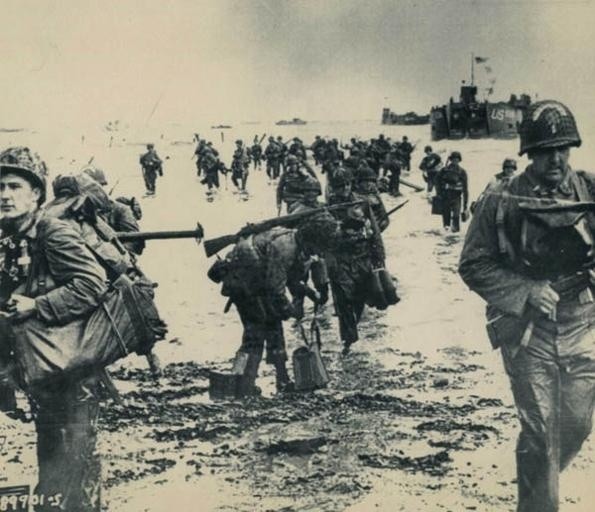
[{"xmin": 292, "ymin": 311, "xmax": 331, "ymax": 391}]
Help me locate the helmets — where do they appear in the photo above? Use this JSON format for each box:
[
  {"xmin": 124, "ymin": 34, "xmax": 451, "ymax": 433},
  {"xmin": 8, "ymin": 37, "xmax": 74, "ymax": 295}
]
[
  {"xmin": 516, "ymin": 98, "xmax": 584, "ymax": 156},
  {"xmin": 502, "ymin": 157, "xmax": 518, "ymax": 170},
  {"xmin": 357, "ymin": 166, "xmax": 377, "ymax": 183},
  {"xmin": 448, "ymin": 151, "xmax": 463, "ymax": 162},
  {"xmin": 284, "ymin": 154, "xmax": 299, "ymax": 166},
  {"xmin": 146, "ymin": 135, "xmax": 408, "ymax": 151},
  {"xmin": 1, "ymin": 144, "xmax": 48, "ymax": 200},
  {"xmin": 51, "ymin": 172, "xmax": 81, "ymax": 195},
  {"xmin": 296, "ymin": 208, "xmax": 343, "ymax": 254},
  {"xmin": 81, "ymin": 166, "xmax": 109, "ymax": 187},
  {"xmin": 329, "ymin": 168, "xmax": 352, "ymax": 186},
  {"xmin": 424, "ymin": 146, "xmax": 433, "ymax": 153}
]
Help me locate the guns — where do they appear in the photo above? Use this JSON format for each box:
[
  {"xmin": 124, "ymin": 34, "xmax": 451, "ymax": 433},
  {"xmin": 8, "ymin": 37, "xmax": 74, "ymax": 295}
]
[
  {"xmin": 487, "ymin": 270, "xmax": 594, "ymax": 351},
  {"xmin": 203, "ymin": 199, "xmax": 364, "ymax": 259}
]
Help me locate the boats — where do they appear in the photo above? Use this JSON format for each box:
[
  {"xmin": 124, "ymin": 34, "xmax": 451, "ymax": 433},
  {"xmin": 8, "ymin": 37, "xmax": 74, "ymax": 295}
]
[
  {"xmin": 275, "ymin": 118, "xmax": 308, "ymax": 128},
  {"xmin": 210, "ymin": 125, "xmax": 232, "ymax": 130},
  {"xmin": 381, "ymin": 97, "xmax": 433, "ymax": 128},
  {"xmin": 426, "ymin": 52, "xmax": 531, "ymax": 145}
]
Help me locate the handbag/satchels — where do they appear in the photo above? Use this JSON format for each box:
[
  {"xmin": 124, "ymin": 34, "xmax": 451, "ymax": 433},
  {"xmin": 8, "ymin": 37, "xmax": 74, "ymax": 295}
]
[
  {"xmin": 367, "ymin": 266, "xmax": 400, "ymax": 310},
  {"xmin": 461, "ymin": 209, "xmax": 470, "ymax": 223},
  {"xmin": 5, "ymin": 274, "xmax": 165, "ymax": 390}
]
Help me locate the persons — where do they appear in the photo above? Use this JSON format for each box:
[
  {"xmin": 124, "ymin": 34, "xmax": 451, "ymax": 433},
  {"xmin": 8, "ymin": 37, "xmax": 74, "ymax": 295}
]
[
  {"xmin": 80, "ymin": 162, "xmax": 160, "ymax": 377},
  {"xmin": 0, "ymin": 144, "xmax": 112, "ymax": 511},
  {"xmin": 207, "ymin": 155, "xmax": 391, "ymax": 398},
  {"xmin": 192, "ymin": 131, "xmax": 469, "ymax": 233},
  {"xmin": 470, "ymin": 158, "xmax": 516, "ymax": 214},
  {"xmin": 459, "ymin": 98, "xmax": 595, "ymax": 511},
  {"xmin": 139, "ymin": 143, "xmax": 164, "ymax": 195}
]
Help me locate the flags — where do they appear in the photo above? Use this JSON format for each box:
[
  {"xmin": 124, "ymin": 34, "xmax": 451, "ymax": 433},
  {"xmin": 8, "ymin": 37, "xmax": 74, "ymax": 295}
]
[{"xmin": 475, "ymin": 55, "xmax": 489, "ymax": 65}]
